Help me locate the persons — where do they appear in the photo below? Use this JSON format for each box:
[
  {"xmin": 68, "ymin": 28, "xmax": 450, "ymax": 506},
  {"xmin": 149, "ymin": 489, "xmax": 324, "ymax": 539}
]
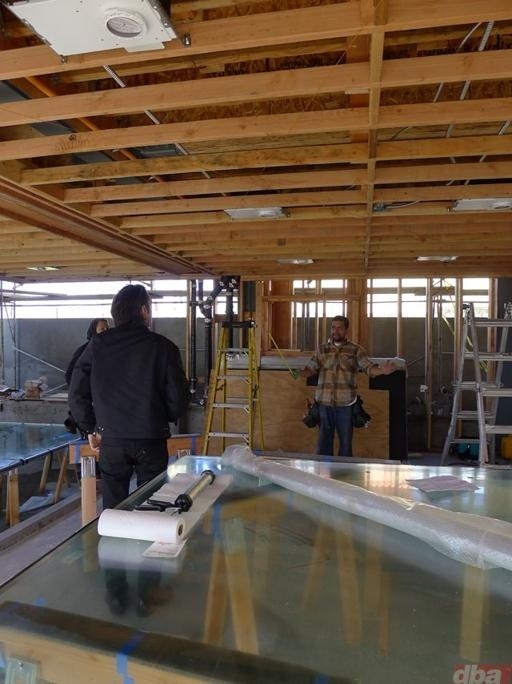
[
  {"xmin": 68, "ymin": 284, "xmax": 192, "ymax": 621},
  {"xmin": 65, "ymin": 318, "xmax": 108, "ymax": 385},
  {"xmin": 300, "ymin": 315, "xmax": 396, "ymax": 457}
]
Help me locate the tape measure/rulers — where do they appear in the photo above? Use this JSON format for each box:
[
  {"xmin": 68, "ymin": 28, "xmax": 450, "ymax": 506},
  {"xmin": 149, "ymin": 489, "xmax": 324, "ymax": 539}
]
[{"xmin": 265, "ymin": 329, "xmax": 301, "ymax": 382}]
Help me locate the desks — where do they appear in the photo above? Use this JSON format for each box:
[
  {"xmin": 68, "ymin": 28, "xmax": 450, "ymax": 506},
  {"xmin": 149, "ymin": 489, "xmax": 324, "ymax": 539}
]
[
  {"xmin": 0, "ymin": 420, "xmax": 86, "ymax": 472},
  {"xmin": 0, "ymin": 454, "xmax": 512, "ymax": 684}
]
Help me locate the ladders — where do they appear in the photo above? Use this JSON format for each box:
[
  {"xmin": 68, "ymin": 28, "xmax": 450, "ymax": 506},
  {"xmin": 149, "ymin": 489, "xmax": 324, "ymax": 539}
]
[
  {"xmin": 201, "ymin": 320, "xmax": 264, "ymax": 456},
  {"xmin": 441, "ymin": 302, "xmax": 512, "ymax": 469}
]
